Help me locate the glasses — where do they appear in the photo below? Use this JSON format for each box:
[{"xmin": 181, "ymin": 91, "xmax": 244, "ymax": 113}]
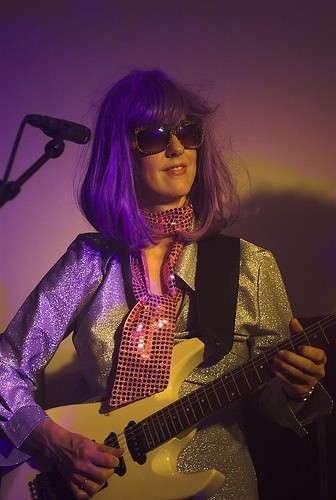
[{"xmin": 128, "ymin": 115, "xmax": 206, "ymax": 158}]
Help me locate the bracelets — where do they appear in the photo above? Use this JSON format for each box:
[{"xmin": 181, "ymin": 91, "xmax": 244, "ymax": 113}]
[{"xmin": 282, "ymin": 384, "xmax": 317, "ymax": 401}]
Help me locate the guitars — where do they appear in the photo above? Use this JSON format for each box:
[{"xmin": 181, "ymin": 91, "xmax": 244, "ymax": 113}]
[{"xmin": 0, "ymin": 312, "xmax": 336, "ymax": 500}]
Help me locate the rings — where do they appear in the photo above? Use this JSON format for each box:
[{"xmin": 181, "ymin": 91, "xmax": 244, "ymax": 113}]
[{"xmin": 81, "ymin": 476, "xmax": 89, "ymax": 488}]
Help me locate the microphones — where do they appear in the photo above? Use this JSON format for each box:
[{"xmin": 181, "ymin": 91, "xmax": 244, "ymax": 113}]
[{"xmin": 24, "ymin": 114, "xmax": 91, "ymax": 144}]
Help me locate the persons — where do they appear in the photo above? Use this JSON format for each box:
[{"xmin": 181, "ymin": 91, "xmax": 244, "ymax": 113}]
[{"xmin": 0, "ymin": 67, "xmax": 325, "ymax": 500}]
